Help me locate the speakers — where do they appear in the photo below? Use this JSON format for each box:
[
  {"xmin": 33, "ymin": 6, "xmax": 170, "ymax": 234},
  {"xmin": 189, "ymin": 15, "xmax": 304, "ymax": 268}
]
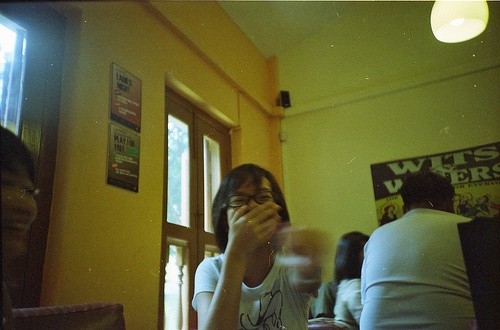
[{"xmin": 279, "ymin": 90, "xmax": 291, "ymax": 108}]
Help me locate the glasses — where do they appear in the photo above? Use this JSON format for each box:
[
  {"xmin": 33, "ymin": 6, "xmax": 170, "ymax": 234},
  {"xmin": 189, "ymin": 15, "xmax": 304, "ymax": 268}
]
[
  {"xmin": 225, "ymin": 191, "xmax": 276, "ymax": 208},
  {"xmin": 0, "ymin": 176, "xmax": 52, "ymax": 208}
]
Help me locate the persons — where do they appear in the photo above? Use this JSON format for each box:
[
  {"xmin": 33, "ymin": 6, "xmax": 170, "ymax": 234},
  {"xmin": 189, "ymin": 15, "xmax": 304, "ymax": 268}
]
[
  {"xmin": 360, "ymin": 171, "xmax": 478, "ymax": 330},
  {"xmin": 333, "ymin": 231, "xmax": 370, "ymax": 330},
  {"xmin": 192, "ymin": 163, "xmax": 326, "ymax": 330},
  {"xmin": 457, "ymin": 195, "xmax": 490, "ymax": 218},
  {"xmin": 315, "ymin": 280, "xmax": 337, "ymax": 318},
  {"xmin": 379, "ymin": 204, "xmax": 398, "ymax": 226},
  {"xmin": 0, "ymin": 126, "xmax": 37, "ymax": 330}
]
